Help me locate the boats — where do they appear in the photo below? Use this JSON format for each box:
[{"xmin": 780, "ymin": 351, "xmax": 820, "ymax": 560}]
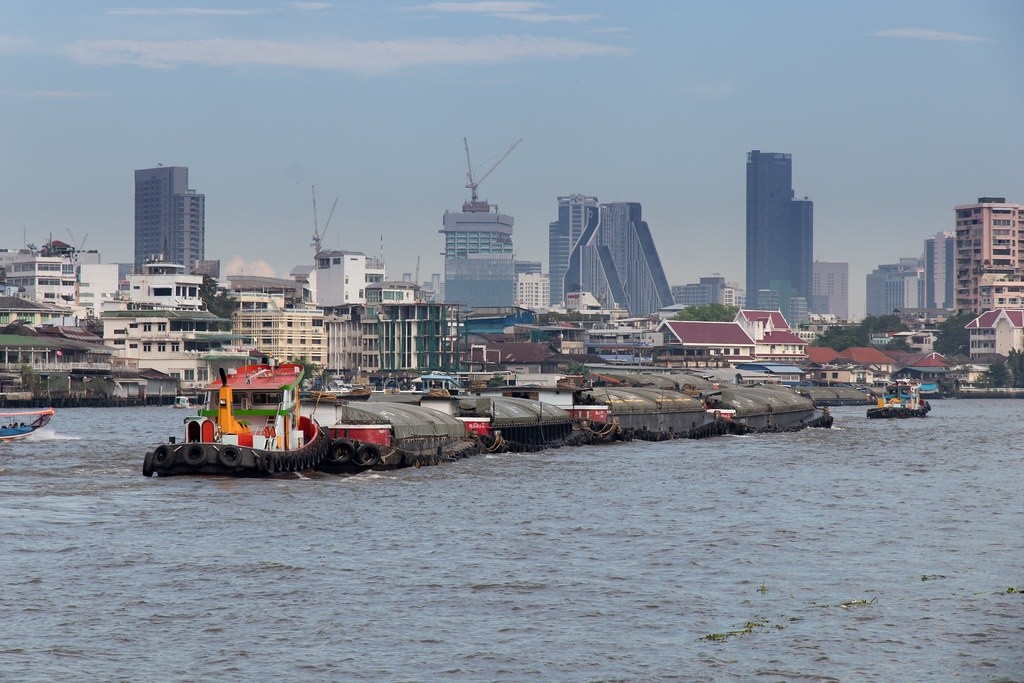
[
  {"xmin": 289, "ymin": 369, "xmax": 462, "ymax": 409},
  {"xmin": 867, "ymin": 378, "xmax": 931, "ymax": 419},
  {"xmin": 143, "ymin": 348, "xmax": 837, "ymax": 480},
  {"xmin": 0, "ymin": 408, "xmax": 54, "ymax": 443},
  {"xmin": 173, "ymin": 396, "xmax": 191, "ymax": 408}
]
